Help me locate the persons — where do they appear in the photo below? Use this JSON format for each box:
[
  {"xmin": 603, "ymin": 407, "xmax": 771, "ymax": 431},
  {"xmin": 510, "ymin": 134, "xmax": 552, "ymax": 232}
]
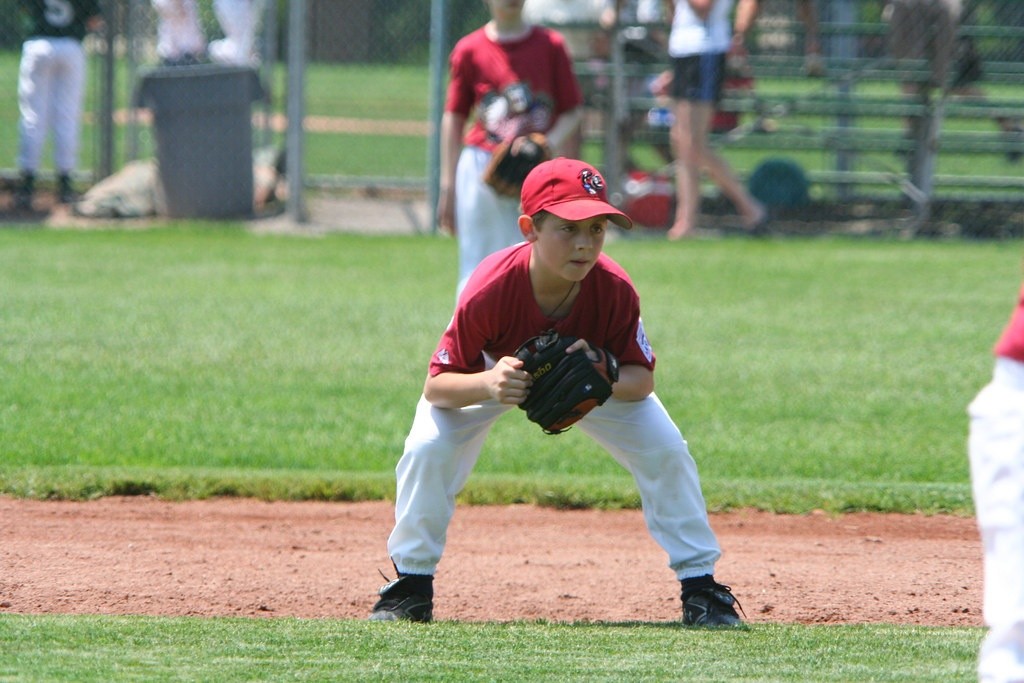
[
  {"xmin": 966, "ymin": 281, "xmax": 1023, "ymax": 683},
  {"xmin": 882, "ymin": 0, "xmax": 957, "ymax": 213},
  {"xmin": 519, "ymin": 0, "xmax": 824, "ymax": 240},
  {"xmin": 369, "ymin": 158, "xmax": 743, "ymax": 624},
  {"xmin": 14, "ymin": 1, "xmax": 270, "ymax": 203},
  {"xmin": 437, "ymin": 0, "xmax": 585, "ymax": 301}
]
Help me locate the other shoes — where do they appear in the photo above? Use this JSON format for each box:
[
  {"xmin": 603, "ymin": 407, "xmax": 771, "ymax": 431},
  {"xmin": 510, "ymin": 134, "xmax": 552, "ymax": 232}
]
[
  {"xmin": 16, "ymin": 182, "xmax": 34, "ymax": 211},
  {"xmin": 57, "ymin": 178, "xmax": 81, "ymax": 205}
]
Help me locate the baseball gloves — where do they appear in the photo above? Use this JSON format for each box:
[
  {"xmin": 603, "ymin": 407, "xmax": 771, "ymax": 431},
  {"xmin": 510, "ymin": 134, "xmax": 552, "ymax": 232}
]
[
  {"xmin": 503, "ymin": 334, "xmax": 610, "ymax": 436},
  {"xmin": 484, "ymin": 131, "xmax": 550, "ymax": 200}
]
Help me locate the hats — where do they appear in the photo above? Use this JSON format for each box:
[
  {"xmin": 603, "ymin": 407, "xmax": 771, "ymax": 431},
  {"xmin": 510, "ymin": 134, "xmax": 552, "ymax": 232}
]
[{"xmin": 521, "ymin": 157, "xmax": 633, "ymax": 230}]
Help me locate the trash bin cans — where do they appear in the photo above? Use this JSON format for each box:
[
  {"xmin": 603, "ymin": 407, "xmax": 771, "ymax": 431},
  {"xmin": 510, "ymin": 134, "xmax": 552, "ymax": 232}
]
[{"xmin": 129, "ymin": 64, "xmax": 268, "ymax": 222}]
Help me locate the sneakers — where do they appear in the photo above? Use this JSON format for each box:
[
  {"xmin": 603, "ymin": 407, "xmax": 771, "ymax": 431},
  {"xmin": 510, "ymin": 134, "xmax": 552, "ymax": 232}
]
[
  {"xmin": 681, "ymin": 574, "xmax": 747, "ymax": 627},
  {"xmin": 370, "ymin": 557, "xmax": 434, "ymax": 623}
]
